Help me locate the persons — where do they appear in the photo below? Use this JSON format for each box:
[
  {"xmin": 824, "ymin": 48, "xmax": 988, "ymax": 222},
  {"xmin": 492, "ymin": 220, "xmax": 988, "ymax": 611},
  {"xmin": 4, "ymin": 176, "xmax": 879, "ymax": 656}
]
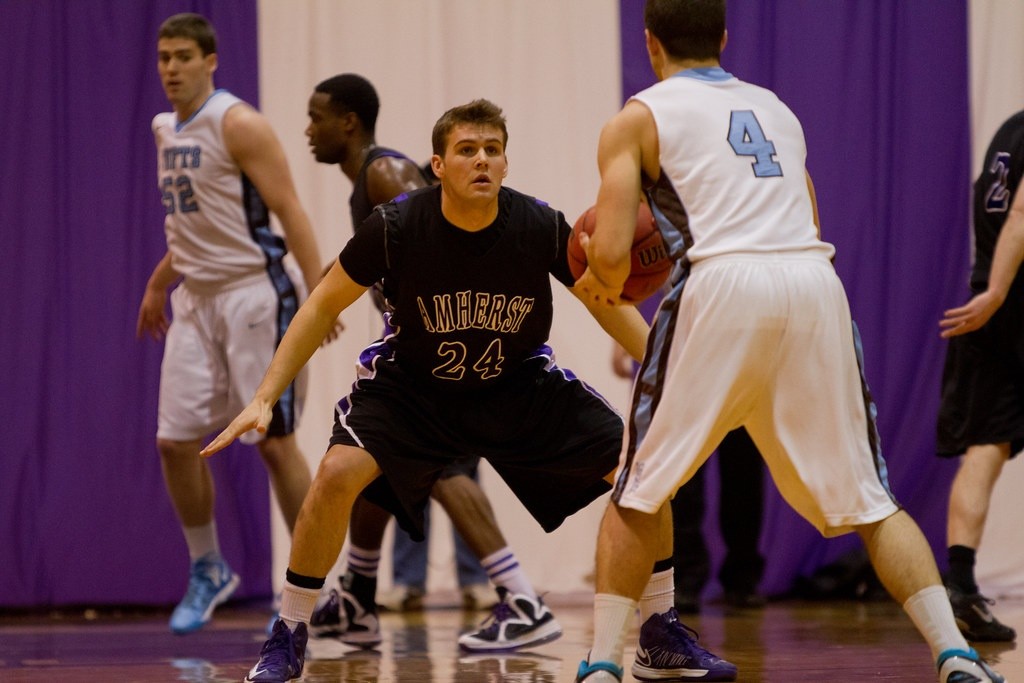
[
  {"xmin": 200, "ymin": 98, "xmax": 738, "ymax": 683},
  {"xmin": 306, "ymin": 70, "xmax": 563, "ymax": 652},
  {"xmin": 573, "ymin": 0, "xmax": 1006, "ymax": 683},
  {"xmin": 135, "ymin": 12, "xmax": 344, "ymax": 644},
  {"xmin": 934, "ymin": 109, "xmax": 1024, "ymax": 641}
]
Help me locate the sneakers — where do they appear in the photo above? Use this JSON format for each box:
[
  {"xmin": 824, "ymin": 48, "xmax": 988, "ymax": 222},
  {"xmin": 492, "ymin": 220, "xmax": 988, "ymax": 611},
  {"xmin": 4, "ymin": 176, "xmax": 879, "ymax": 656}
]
[
  {"xmin": 457, "ymin": 585, "xmax": 564, "ymax": 653},
  {"xmin": 244, "ymin": 617, "xmax": 309, "ymax": 683},
  {"xmin": 169, "ymin": 549, "xmax": 241, "ymax": 634},
  {"xmin": 267, "ymin": 582, "xmax": 382, "ymax": 648},
  {"xmin": 945, "ymin": 586, "xmax": 1017, "ymax": 643},
  {"xmin": 630, "ymin": 604, "xmax": 738, "ymax": 683},
  {"xmin": 573, "ymin": 648, "xmax": 624, "ymax": 683},
  {"xmin": 936, "ymin": 646, "xmax": 1004, "ymax": 683}
]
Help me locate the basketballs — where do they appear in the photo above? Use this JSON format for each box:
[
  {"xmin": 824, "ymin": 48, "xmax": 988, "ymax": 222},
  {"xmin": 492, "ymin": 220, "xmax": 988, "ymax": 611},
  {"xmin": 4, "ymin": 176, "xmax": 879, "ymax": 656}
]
[{"xmin": 567, "ymin": 195, "xmax": 671, "ymax": 306}]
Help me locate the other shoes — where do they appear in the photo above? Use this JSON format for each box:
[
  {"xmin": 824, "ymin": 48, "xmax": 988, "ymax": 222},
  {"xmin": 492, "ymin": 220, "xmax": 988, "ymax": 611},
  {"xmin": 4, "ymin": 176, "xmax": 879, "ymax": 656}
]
[
  {"xmin": 459, "ymin": 586, "xmax": 486, "ymax": 630},
  {"xmin": 376, "ymin": 579, "xmax": 424, "ymax": 612}
]
